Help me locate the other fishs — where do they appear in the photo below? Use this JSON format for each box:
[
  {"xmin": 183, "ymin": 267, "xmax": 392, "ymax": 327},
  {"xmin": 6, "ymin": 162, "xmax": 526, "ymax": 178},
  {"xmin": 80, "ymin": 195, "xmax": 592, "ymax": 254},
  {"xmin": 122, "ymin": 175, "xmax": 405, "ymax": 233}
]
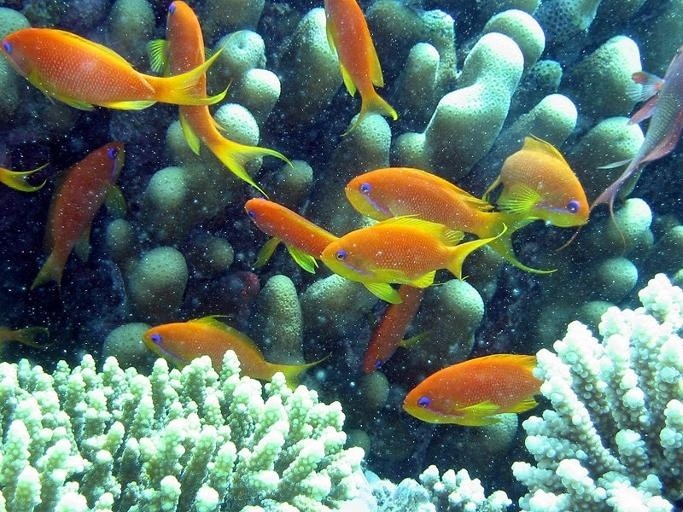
[{"xmin": 0, "ymin": 0, "xmax": 590, "ymax": 431}]
